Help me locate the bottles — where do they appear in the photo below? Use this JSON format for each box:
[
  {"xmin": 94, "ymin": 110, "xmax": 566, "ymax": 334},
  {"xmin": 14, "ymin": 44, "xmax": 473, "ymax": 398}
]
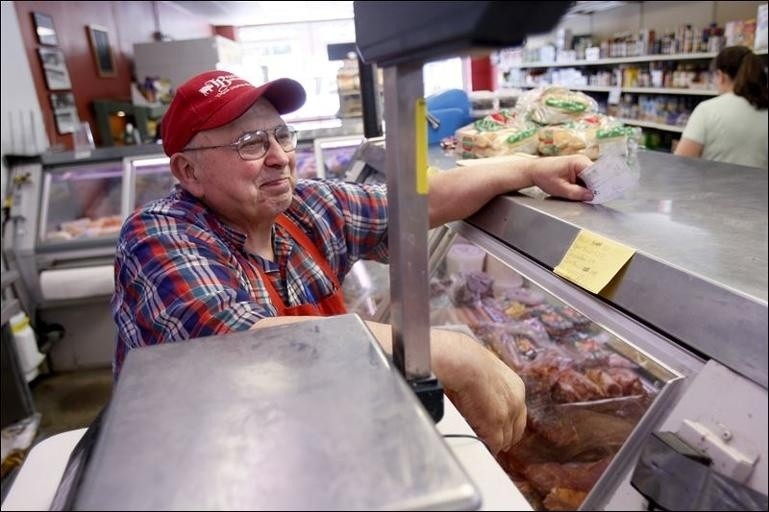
[{"xmin": 607, "ymin": 22, "xmax": 726, "ymax": 126}]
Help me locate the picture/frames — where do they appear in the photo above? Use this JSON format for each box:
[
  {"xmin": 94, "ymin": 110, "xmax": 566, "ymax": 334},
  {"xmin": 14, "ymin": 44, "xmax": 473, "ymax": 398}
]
[{"xmin": 29, "ymin": 9, "xmax": 119, "ymax": 137}]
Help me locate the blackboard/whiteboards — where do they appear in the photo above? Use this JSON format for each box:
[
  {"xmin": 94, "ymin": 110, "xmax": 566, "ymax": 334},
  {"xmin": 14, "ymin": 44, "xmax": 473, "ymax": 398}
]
[{"xmin": 87, "ymin": 24, "xmax": 118, "ymax": 77}]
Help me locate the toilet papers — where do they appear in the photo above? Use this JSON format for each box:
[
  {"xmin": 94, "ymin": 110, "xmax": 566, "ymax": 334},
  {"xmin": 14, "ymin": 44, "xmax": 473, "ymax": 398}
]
[{"xmin": 40, "ymin": 266, "xmax": 116, "ymax": 301}]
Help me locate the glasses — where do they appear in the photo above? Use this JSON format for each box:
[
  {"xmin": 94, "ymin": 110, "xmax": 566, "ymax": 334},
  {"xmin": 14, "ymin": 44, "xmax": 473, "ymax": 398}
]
[{"xmin": 180, "ymin": 124, "xmax": 299, "ymax": 162}]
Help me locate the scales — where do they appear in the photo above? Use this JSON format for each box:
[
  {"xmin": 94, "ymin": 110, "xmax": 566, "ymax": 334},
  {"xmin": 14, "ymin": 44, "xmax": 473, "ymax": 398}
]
[{"xmin": 48, "ymin": 3, "xmax": 576, "ymax": 511}]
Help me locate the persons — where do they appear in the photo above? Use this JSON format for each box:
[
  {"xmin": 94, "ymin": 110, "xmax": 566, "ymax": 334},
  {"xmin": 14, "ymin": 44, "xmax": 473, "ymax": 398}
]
[
  {"xmin": 110, "ymin": 67, "xmax": 597, "ymax": 458},
  {"xmin": 669, "ymin": 44, "xmax": 768, "ymax": 169}
]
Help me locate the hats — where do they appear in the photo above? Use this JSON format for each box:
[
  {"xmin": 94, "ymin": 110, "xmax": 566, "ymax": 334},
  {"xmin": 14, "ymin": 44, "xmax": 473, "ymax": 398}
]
[{"xmin": 161, "ymin": 68, "xmax": 308, "ymax": 158}]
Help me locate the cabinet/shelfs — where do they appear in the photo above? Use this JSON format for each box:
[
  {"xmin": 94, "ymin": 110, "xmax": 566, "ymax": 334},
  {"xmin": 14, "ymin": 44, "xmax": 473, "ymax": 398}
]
[
  {"xmin": 496, "ymin": 47, "xmax": 769, "ymax": 136},
  {"xmin": 336, "ymin": 136, "xmax": 769, "ymax": 512},
  {"xmin": 31, "ymin": 133, "xmax": 385, "ymax": 309}
]
